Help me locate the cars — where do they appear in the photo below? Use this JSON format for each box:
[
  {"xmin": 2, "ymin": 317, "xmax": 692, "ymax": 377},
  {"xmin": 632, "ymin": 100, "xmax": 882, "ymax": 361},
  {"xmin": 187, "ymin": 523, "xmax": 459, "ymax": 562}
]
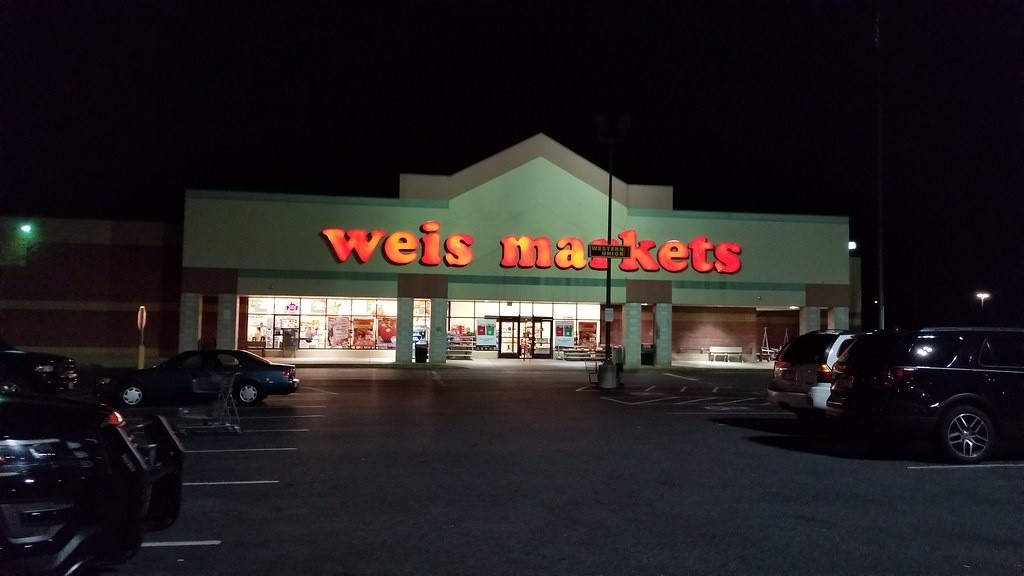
[
  {"xmin": 0, "ymin": 339, "xmax": 80, "ymax": 402},
  {"xmin": 92, "ymin": 348, "xmax": 303, "ymax": 409},
  {"xmin": 0, "ymin": 389, "xmax": 186, "ymax": 576}
]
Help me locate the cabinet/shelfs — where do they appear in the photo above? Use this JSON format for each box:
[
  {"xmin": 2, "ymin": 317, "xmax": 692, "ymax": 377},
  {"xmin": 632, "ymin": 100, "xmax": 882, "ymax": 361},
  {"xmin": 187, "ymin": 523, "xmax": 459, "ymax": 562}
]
[
  {"xmin": 564, "ymin": 348, "xmax": 606, "ymax": 361},
  {"xmin": 445, "ymin": 335, "xmax": 477, "ymax": 360}
]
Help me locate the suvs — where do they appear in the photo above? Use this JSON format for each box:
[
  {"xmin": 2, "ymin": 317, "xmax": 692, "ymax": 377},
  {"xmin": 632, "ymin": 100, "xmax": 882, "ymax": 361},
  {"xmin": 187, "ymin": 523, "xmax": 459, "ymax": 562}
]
[
  {"xmin": 827, "ymin": 325, "xmax": 1024, "ymax": 464},
  {"xmin": 766, "ymin": 327, "xmax": 872, "ymax": 426}
]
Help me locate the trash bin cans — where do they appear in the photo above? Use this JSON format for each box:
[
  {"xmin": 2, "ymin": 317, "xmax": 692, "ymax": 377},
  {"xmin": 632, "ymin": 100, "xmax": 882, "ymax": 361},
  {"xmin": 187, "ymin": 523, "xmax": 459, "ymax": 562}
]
[
  {"xmin": 612, "ymin": 345, "xmax": 624, "ymax": 366},
  {"xmin": 641, "ymin": 343, "xmax": 655, "ymax": 365},
  {"xmin": 415, "ymin": 341, "xmax": 428, "ymax": 363}
]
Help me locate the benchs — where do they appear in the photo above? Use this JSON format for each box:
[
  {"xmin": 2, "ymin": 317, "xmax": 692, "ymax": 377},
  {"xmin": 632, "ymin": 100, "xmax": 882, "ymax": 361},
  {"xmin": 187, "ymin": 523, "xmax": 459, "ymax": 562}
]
[{"xmin": 709, "ymin": 346, "xmax": 744, "ymax": 362}]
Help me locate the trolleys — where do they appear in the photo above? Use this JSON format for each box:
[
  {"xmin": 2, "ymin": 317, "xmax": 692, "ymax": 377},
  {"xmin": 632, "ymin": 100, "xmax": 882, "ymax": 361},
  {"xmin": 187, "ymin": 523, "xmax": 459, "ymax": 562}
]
[{"xmin": 166, "ymin": 371, "xmax": 243, "ymax": 442}]
